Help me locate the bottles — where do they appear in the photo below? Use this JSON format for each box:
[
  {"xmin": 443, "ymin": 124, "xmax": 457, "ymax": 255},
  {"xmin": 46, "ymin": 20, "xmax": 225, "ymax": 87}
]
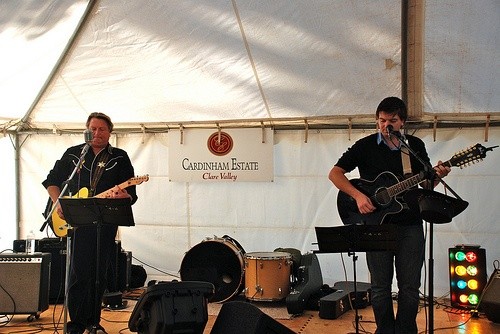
[{"xmin": 26, "ymin": 230, "xmax": 35, "ymax": 254}]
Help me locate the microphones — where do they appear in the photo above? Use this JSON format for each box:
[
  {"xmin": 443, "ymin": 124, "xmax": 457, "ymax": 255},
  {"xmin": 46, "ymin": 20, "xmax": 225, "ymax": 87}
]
[
  {"xmin": 81, "ymin": 129, "xmax": 93, "ymax": 154},
  {"xmin": 386, "ymin": 125, "xmax": 393, "ymax": 136}
]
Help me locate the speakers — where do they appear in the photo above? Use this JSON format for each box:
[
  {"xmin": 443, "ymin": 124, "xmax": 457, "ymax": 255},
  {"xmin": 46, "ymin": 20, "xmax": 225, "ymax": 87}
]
[
  {"xmin": 210, "ymin": 302, "xmax": 296, "ymax": 334},
  {"xmin": 0, "ymin": 252, "xmax": 51, "ymax": 315},
  {"xmin": 128, "ymin": 281, "xmax": 215, "ymax": 334},
  {"xmin": 480, "ymin": 269, "xmax": 500, "ymax": 323}
]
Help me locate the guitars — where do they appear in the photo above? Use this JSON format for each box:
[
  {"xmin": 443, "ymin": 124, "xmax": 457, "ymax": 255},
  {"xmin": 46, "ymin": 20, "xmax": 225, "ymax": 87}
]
[
  {"xmin": 336, "ymin": 143, "xmax": 499, "ymax": 226},
  {"xmin": 51, "ymin": 175, "xmax": 148, "ymax": 237}
]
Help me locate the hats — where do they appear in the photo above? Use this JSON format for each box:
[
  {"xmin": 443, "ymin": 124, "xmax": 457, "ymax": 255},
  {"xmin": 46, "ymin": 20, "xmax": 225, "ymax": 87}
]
[{"xmin": 404, "ymin": 188, "xmax": 469, "ymax": 223}]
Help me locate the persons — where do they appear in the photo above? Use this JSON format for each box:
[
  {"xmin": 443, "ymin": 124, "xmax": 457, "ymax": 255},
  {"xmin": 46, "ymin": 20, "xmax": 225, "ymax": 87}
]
[
  {"xmin": 42, "ymin": 112, "xmax": 138, "ymax": 334},
  {"xmin": 328, "ymin": 97, "xmax": 451, "ymax": 334}
]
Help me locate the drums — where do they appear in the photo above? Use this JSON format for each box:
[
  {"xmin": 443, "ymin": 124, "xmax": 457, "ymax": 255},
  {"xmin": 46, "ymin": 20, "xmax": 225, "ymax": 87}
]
[
  {"xmin": 245, "ymin": 252, "xmax": 290, "ymax": 302},
  {"xmin": 180, "ymin": 236, "xmax": 246, "ymax": 304}
]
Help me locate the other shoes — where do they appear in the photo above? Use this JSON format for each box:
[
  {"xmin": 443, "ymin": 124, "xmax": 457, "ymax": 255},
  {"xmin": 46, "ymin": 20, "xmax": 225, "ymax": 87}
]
[{"xmin": 87, "ymin": 324, "xmax": 105, "ymax": 331}]
